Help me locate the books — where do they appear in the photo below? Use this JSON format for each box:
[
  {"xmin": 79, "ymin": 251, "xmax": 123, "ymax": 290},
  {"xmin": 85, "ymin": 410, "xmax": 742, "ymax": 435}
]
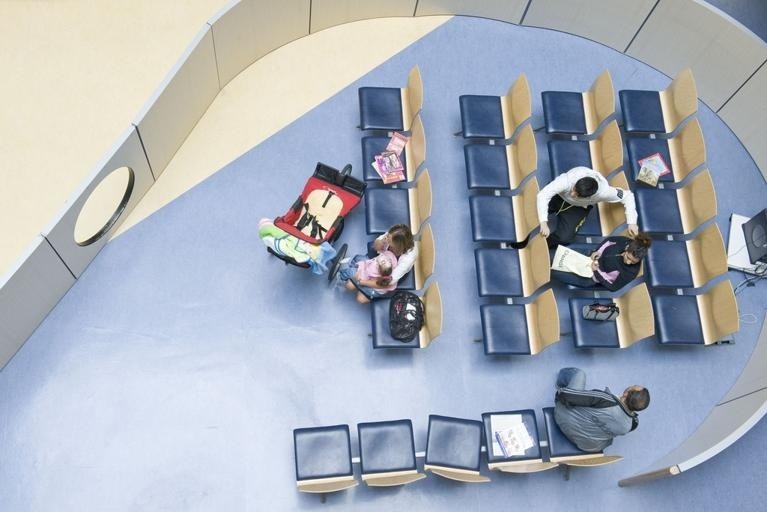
[
  {"xmin": 637, "ymin": 152, "xmax": 671, "ymax": 187},
  {"xmin": 371, "ymin": 132, "xmax": 409, "ymax": 185}
]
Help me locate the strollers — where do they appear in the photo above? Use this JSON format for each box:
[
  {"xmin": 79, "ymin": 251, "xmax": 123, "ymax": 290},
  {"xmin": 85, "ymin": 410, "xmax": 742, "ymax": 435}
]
[{"xmin": 256, "ymin": 160, "xmax": 367, "ymax": 282}]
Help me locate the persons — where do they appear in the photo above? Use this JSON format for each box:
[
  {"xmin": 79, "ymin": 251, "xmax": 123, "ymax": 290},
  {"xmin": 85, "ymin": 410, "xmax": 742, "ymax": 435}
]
[
  {"xmin": 555, "ymin": 368, "xmax": 650, "ymax": 453},
  {"xmin": 345, "ymin": 224, "xmax": 418, "ymax": 303},
  {"xmin": 536, "ymin": 166, "xmax": 639, "ymax": 238},
  {"xmin": 336, "ymin": 250, "xmax": 397, "ymax": 286},
  {"xmin": 551, "ymin": 233, "xmax": 652, "ymax": 291}
]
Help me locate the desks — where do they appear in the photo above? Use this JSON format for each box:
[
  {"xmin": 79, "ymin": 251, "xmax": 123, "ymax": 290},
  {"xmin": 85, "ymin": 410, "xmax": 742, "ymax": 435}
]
[{"xmin": 726, "ymin": 214, "xmax": 766, "ymax": 278}]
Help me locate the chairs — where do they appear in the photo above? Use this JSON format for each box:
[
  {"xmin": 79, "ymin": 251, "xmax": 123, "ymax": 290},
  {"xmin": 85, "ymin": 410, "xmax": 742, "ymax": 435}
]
[
  {"xmin": 634, "ymin": 169, "xmax": 717, "ymax": 234},
  {"xmin": 365, "ymin": 169, "xmax": 432, "ymax": 235},
  {"xmin": 576, "ymin": 170, "xmax": 630, "ymax": 238},
  {"xmin": 358, "ymin": 66, "xmax": 423, "ymax": 131},
  {"xmin": 548, "ymin": 119, "xmax": 623, "ymax": 181},
  {"xmin": 534, "ymin": 68, "xmax": 616, "ymax": 135},
  {"xmin": 543, "ymin": 407, "xmax": 624, "ymax": 480},
  {"xmin": 482, "ymin": 409, "xmax": 560, "ymax": 474},
  {"xmin": 469, "ymin": 176, "xmax": 540, "ymax": 243},
  {"xmin": 626, "ymin": 118, "xmax": 705, "ymax": 182},
  {"xmin": 358, "ymin": 419, "xmax": 427, "ymax": 487},
  {"xmin": 619, "ymin": 79, "xmax": 697, "ymax": 133},
  {"xmin": 644, "ymin": 223, "xmax": 728, "ymax": 289},
  {"xmin": 367, "ymin": 223, "xmax": 435, "ymax": 291},
  {"xmin": 474, "ymin": 232, "xmax": 551, "ymax": 298},
  {"xmin": 372, "ymin": 281, "xmax": 443, "ymax": 348},
  {"xmin": 568, "ymin": 228, "xmax": 643, "ymax": 290},
  {"xmin": 454, "ymin": 73, "xmax": 532, "ymax": 138},
  {"xmin": 293, "ymin": 424, "xmax": 359, "ymax": 493},
  {"xmin": 480, "ymin": 288, "xmax": 561, "ymax": 355},
  {"xmin": 464, "ymin": 123, "xmax": 537, "ymax": 190},
  {"xmin": 653, "ymin": 280, "xmax": 739, "ymax": 346},
  {"xmin": 424, "ymin": 415, "xmax": 491, "ymax": 483},
  {"xmin": 361, "ymin": 115, "xmax": 426, "ymax": 182},
  {"xmin": 568, "ymin": 281, "xmax": 655, "ymax": 348}
]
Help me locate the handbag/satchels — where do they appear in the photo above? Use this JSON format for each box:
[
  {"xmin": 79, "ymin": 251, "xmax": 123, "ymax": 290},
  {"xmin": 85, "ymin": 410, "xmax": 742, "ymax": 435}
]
[
  {"xmin": 390, "ymin": 290, "xmax": 424, "ymax": 343},
  {"xmin": 581, "ymin": 301, "xmax": 620, "ymax": 321},
  {"xmin": 550, "ymin": 244, "xmax": 595, "ymax": 280}
]
